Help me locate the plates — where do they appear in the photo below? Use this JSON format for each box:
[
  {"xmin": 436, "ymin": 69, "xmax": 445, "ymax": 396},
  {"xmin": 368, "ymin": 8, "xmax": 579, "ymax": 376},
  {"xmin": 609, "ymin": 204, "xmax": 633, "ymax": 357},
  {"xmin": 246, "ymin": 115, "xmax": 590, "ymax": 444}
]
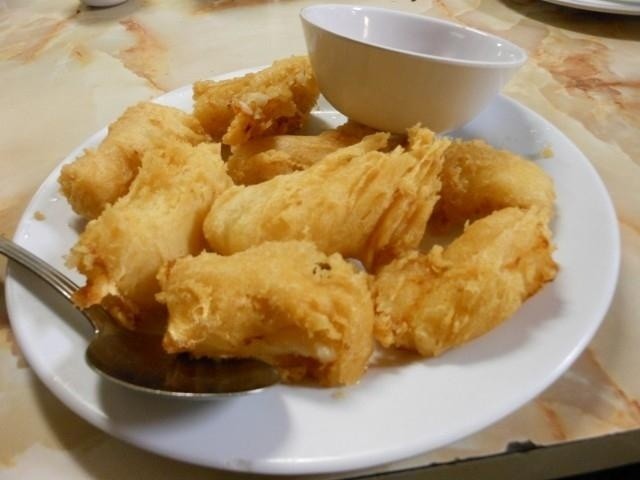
[{"xmin": 3, "ymin": 69, "xmax": 621, "ymax": 476}]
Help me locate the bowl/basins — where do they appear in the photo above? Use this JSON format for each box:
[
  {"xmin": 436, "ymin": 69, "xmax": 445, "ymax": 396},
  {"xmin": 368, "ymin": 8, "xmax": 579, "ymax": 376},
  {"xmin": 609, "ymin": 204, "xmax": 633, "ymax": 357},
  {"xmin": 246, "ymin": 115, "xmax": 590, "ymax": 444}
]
[{"xmin": 301, "ymin": 3, "xmax": 527, "ymax": 135}]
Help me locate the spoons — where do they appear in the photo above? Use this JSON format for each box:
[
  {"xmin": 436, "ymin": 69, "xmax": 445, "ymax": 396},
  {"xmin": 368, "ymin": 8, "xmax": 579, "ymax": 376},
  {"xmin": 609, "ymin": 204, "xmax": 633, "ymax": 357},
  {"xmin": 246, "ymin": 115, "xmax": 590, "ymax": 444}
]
[{"xmin": 3, "ymin": 235, "xmax": 281, "ymax": 400}]
